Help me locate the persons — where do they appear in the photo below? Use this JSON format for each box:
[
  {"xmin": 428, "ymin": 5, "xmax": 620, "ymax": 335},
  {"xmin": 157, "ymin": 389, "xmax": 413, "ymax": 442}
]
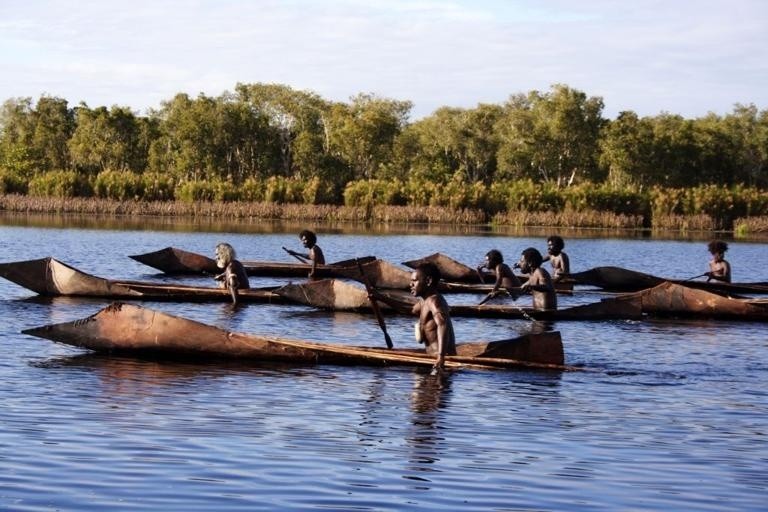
[
  {"xmin": 488, "ymin": 247, "xmax": 557, "ymax": 309},
  {"xmin": 519, "ymin": 236, "xmax": 569, "ymax": 278},
  {"xmin": 708, "ymin": 240, "xmax": 731, "ymax": 283},
  {"xmin": 365, "ymin": 263, "xmax": 456, "ymax": 372},
  {"xmin": 214, "ymin": 243, "xmax": 250, "ymax": 309},
  {"xmin": 288, "ymin": 230, "xmax": 325, "ymax": 277},
  {"xmin": 478, "ymin": 249, "xmax": 523, "ymax": 290}
]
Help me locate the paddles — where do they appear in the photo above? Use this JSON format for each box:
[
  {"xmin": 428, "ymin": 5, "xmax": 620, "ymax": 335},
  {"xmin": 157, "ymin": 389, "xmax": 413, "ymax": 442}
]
[{"xmin": 353, "ymin": 256, "xmax": 401, "ymax": 347}]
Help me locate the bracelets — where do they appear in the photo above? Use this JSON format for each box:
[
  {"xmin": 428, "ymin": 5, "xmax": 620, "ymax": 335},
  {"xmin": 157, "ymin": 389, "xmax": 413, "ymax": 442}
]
[{"xmin": 438, "ymin": 352, "xmax": 444, "ymax": 356}]
